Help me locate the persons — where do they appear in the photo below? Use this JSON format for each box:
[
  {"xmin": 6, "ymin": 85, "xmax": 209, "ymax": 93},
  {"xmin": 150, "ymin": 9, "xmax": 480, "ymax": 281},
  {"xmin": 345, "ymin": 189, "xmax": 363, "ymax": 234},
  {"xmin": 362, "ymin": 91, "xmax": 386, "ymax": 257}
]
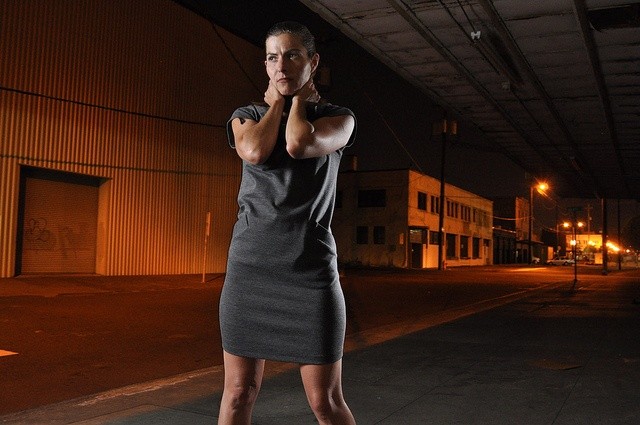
[{"xmin": 216, "ymin": 21, "xmax": 357, "ymax": 425}]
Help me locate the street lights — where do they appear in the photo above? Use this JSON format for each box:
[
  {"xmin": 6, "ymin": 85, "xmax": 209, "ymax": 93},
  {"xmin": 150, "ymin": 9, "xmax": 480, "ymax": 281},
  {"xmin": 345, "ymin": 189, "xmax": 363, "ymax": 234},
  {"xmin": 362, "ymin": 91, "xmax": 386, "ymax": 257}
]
[
  {"xmin": 528, "ymin": 183, "xmax": 546, "ymax": 265},
  {"xmin": 432, "ymin": 118, "xmax": 458, "ymax": 271},
  {"xmin": 564, "ymin": 222, "xmax": 583, "ymax": 289}
]
